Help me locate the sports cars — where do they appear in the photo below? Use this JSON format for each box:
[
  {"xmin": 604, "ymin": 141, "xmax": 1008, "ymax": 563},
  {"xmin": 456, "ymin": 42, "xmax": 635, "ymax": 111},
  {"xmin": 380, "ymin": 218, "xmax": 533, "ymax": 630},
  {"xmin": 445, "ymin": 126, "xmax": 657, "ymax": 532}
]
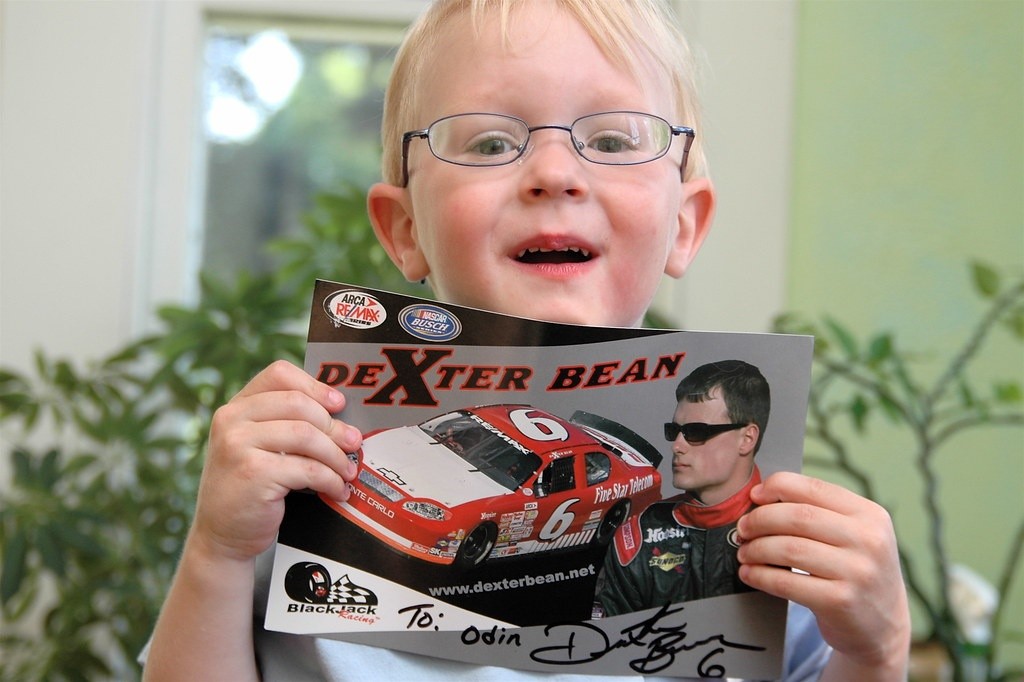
[{"xmin": 316, "ymin": 403, "xmax": 663, "ymax": 570}]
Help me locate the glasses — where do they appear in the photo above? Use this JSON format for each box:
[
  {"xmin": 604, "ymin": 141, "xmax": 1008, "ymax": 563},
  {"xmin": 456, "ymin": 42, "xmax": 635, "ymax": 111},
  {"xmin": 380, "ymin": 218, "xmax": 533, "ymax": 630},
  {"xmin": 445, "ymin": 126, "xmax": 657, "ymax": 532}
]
[
  {"xmin": 402, "ymin": 110, "xmax": 696, "ymax": 188},
  {"xmin": 664, "ymin": 422, "xmax": 748, "ymax": 442}
]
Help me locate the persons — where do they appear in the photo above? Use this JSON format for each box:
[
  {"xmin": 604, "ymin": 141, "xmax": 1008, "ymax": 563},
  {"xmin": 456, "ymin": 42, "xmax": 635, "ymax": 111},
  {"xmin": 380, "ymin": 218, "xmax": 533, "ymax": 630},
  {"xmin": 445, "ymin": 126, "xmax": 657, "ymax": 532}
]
[
  {"xmin": 592, "ymin": 360, "xmax": 770, "ymax": 618},
  {"xmin": 137, "ymin": 0, "xmax": 912, "ymax": 682}
]
